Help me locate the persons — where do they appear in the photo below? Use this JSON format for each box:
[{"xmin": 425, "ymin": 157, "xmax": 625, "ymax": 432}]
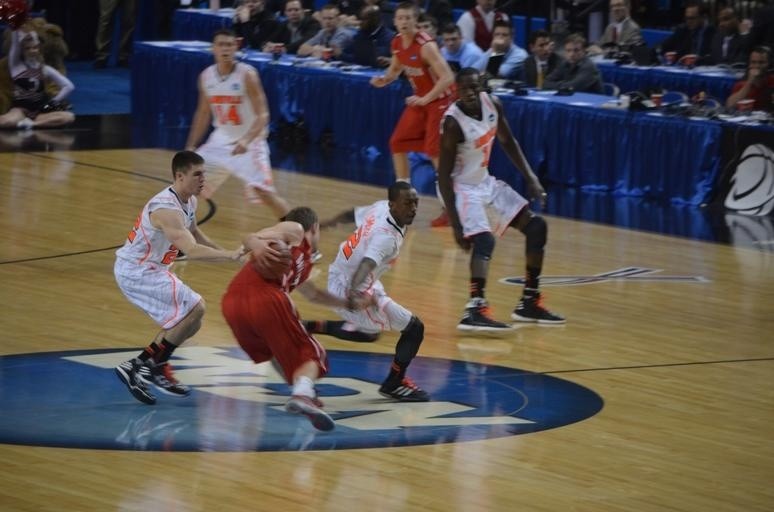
[
  {"xmin": 113, "ymin": 150, "xmax": 251, "ymax": 406},
  {"xmin": 220, "ymin": 206, "xmax": 337, "ymax": 431},
  {"xmin": 2, "ymin": 1, "xmax": 771, "ymax": 129},
  {"xmin": 437, "ymin": 67, "xmax": 567, "ymax": 332},
  {"xmin": 172, "ymin": 29, "xmax": 323, "ymax": 270},
  {"xmin": 369, "ymin": 2, "xmax": 459, "ymax": 226},
  {"xmin": 296, "ymin": 181, "xmax": 432, "ymax": 402}
]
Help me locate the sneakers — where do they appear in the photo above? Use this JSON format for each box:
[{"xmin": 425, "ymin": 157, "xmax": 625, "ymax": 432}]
[
  {"xmin": 287, "ymin": 396, "xmax": 334, "ymax": 432},
  {"xmin": 431, "ymin": 208, "xmax": 452, "ymax": 226},
  {"xmin": 116, "ymin": 358, "xmax": 156, "ymax": 406},
  {"xmin": 457, "ymin": 298, "xmax": 512, "ymax": 337},
  {"xmin": 511, "ymin": 288, "xmax": 565, "ymax": 323},
  {"xmin": 136, "ymin": 360, "xmax": 191, "ymax": 397},
  {"xmin": 117, "ymin": 61, "xmax": 130, "ymax": 68},
  {"xmin": 94, "ymin": 57, "xmax": 107, "ymax": 70},
  {"xmin": 380, "ymin": 378, "xmax": 430, "ymax": 402}
]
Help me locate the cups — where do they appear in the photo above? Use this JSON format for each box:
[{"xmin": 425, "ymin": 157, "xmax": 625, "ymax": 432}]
[
  {"xmin": 684, "ymin": 55, "xmax": 696, "ymax": 65},
  {"xmin": 665, "ymin": 52, "xmax": 678, "ymax": 64},
  {"xmin": 236, "ymin": 37, "xmax": 243, "ymax": 48},
  {"xmin": 652, "ymin": 94, "xmax": 662, "ymax": 108},
  {"xmin": 736, "ymin": 100, "xmax": 755, "ymax": 111},
  {"xmin": 275, "ymin": 42, "xmax": 284, "ymax": 49},
  {"xmin": 323, "ymin": 49, "xmax": 333, "ymax": 59}
]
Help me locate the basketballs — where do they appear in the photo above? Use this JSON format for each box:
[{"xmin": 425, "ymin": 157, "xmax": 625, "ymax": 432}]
[{"xmin": 251, "ymin": 239, "xmax": 293, "ymax": 280}]
